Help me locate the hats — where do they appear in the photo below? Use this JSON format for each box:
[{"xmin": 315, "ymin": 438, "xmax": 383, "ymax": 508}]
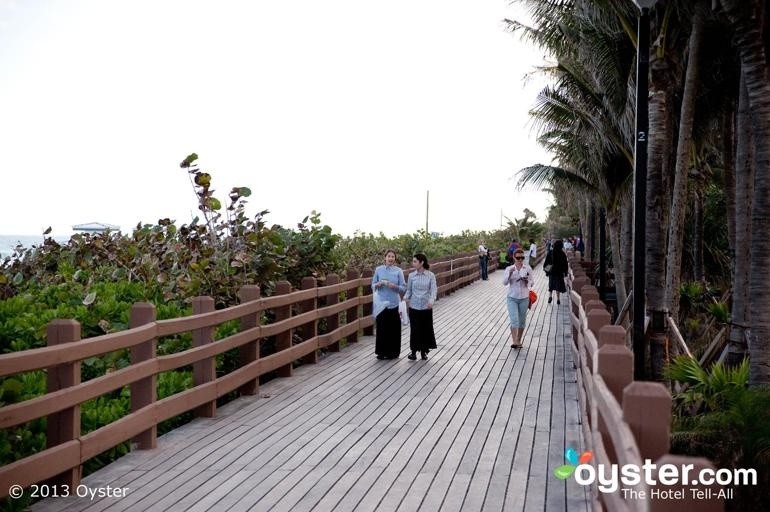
[{"xmin": 529, "ymin": 290, "xmax": 538, "ymax": 310}]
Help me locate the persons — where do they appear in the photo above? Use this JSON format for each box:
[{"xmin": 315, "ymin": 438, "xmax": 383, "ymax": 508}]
[
  {"xmin": 404, "ymin": 254, "xmax": 437, "ymax": 360},
  {"xmin": 503, "ymin": 248, "xmax": 535, "ymax": 348},
  {"xmin": 528, "ymin": 239, "xmax": 536, "ymax": 269},
  {"xmin": 509, "ymin": 238, "xmax": 520, "ymax": 263},
  {"xmin": 478, "ymin": 239, "xmax": 490, "ymax": 280},
  {"xmin": 543, "ymin": 239, "xmax": 568, "ymax": 304},
  {"xmin": 371, "ymin": 250, "xmax": 406, "ymax": 359},
  {"xmin": 499, "ymin": 248, "xmax": 510, "ymax": 269}
]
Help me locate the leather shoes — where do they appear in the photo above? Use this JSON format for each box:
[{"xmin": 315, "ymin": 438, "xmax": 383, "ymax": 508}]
[
  {"xmin": 548, "ymin": 297, "xmax": 553, "ymax": 303},
  {"xmin": 408, "ymin": 350, "xmax": 417, "ymax": 360},
  {"xmin": 376, "ymin": 354, "xmax": 385, "ymax": 360},
  {"xmin": 511, "ymin": 343, "xmax": 523, "ymax": 349},
  {"xmin": 557, "ymin": 299, "xmax": 560, "ymax": 304},
  {"xmin": 388, "ymin": 353, "xmax": 399, "ymax": 357},
  {"xmin": 421, "ymin": 351, "xmax": 428, "ymax": 361}
]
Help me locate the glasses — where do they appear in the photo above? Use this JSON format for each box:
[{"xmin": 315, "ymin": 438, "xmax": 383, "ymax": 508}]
[{"xmin": 515, "ymin": 256, "xmax": 525, "ymax": 260}]
